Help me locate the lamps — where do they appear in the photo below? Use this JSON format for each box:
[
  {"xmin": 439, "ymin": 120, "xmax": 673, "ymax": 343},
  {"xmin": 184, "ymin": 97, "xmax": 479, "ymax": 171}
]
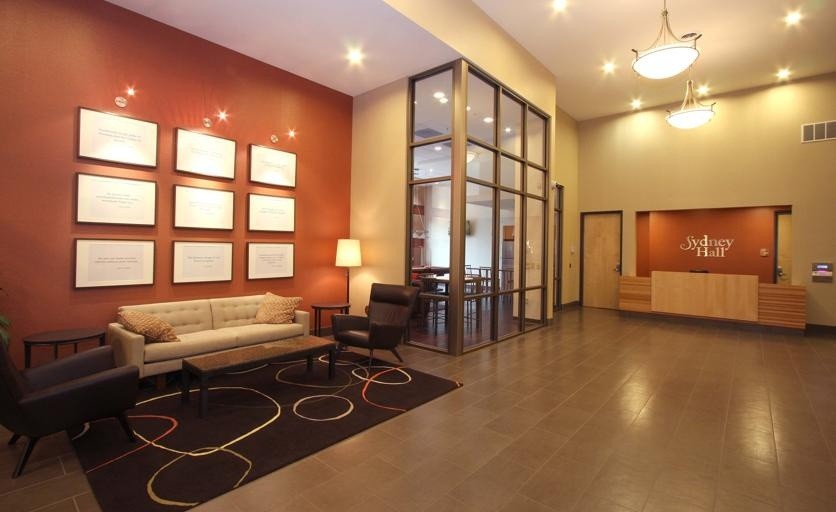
[
  {"xmin": 628, "ymin": 1, "xmax": 704, "ymax": 84},
  {"xmin": 466, "ymin": 149, "xmax": 481, "ymax": 166},
  {"xmin": 664, "ymin": 78, "xmax": 718, "ymax": 130},
  {"xmin": 334, "ymin": 237, "xmax": 363, "ymax": 316}
]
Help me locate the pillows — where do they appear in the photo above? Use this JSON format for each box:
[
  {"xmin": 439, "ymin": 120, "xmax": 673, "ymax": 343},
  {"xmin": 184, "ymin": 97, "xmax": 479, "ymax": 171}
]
[
  {"xmin": 251, "ymin": 291, "xmax": 303, "ymax": 325},
  {"xmin": 115, "ymin": 307, "xmax": 181, "ymax": 343}
]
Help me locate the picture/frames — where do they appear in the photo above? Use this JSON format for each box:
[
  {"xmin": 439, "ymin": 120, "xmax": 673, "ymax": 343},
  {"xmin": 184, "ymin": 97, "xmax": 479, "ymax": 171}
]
[
  {"xmin": 247, "ymin": 193, "xmax": 296, "ymax": 232},
  {"xmin": 172, "ymin": 184, "xmax": 235, "ymax": 231},
  {"xmin": 246, "ymin": 242, "xmax": 295, "ymax": 281},
  {"xmin": 75, "ymin": 172, "xmax": 157, "ymax": 227},
  {"xmin": 74, "ymin": 238, "xmax": 156, "ymax": 289},
  {"xmin": 247, "ymin": 143, "xmax": 296, "ymax": 189},
  {"xmin": 172, "ymin": 240, "xmax": 233, "ymax": 285},
  {"xmin": 77, "ymin": 106, "xmax": 158, "ymax": 169},
  {"xmin": 174, "ymin": 127, "xmax": 237, "ymax": 180}
]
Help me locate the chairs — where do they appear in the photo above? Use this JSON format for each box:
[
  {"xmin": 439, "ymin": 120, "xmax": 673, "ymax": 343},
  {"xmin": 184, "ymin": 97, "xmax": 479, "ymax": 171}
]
[
  {"xmin": 327, "ymin": 281, "xmax": 422, "ymax": 373},
  {"xmin": 0, "ymin": 334, "xmax": 143, "ymax": 479},
  {"xmin": 410, "ymin": 260, "xmax": 516, "ymax": 334}
]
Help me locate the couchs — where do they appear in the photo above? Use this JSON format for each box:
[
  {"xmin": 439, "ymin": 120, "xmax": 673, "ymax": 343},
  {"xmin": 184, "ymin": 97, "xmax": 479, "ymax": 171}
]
[{"xmin": 108, "ymin": 294, "xmax": 312, "ymax": 397}]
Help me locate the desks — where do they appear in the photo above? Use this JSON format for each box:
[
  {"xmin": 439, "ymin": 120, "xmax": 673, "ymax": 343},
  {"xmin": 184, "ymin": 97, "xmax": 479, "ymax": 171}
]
[
  {"xmin": 311, "ymin": 302, "xmax": 352, "ymax": 336},
  {"xmin": 21, "ymin": 326, "xmax": 105, "ymax": 369}
]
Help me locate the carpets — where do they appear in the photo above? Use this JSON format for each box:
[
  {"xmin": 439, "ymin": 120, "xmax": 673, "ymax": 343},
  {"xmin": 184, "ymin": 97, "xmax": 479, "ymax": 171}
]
[{"xmin": 64, "ymin": 347, "xmax": 467, "ymax": 512}]
